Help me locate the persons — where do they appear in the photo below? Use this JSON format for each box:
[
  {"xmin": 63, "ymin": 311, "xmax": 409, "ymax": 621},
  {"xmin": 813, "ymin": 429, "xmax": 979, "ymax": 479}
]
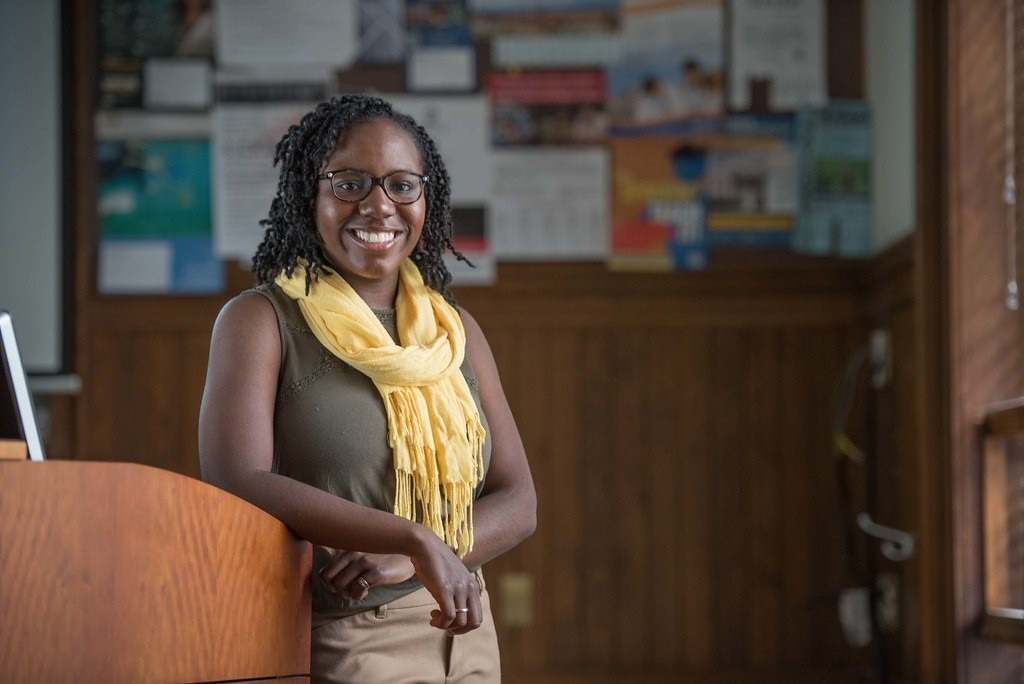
[
  {"xmin": 199, "ymin": 94, "xmax": 537, "ymax": 684},
  {"xmin": 633, "ymin": 59, "xmax": 725, "ymax": 125}
]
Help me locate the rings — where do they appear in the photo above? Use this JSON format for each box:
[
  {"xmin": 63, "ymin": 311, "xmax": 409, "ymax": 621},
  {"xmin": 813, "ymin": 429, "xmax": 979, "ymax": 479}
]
[
  {"xmin": 358, "ymin": 577, "xmax": 369, "ymax": 590},
  {"xmin": 456, "ymin": 609, "xmax": 468, "ymax": 613}
]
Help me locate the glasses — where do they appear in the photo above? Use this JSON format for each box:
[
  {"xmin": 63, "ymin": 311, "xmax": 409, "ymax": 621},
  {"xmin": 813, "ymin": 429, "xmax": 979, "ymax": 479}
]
[{"xmin": 318, "ymin": 167, "xmax": 430, "ymax": 204}]
